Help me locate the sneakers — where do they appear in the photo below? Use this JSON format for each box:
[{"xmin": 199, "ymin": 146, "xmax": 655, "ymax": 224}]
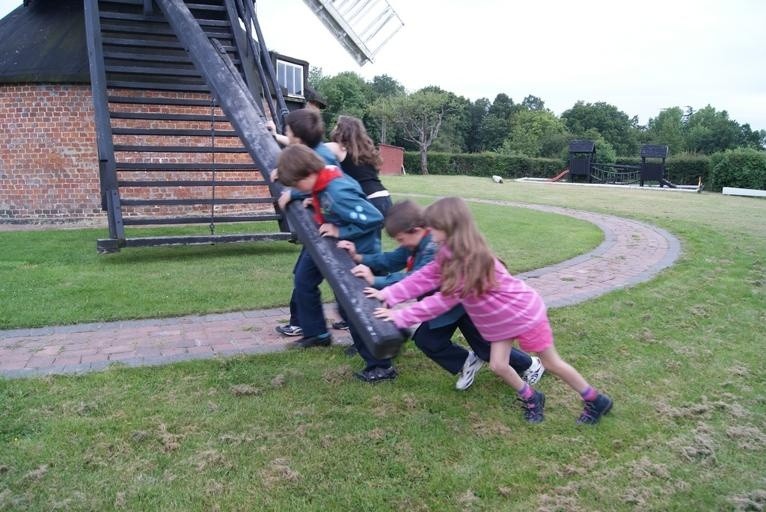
[
  {"xmin": 353, "ymin": 366, "xmax": 397, "ymax": 383},
  {"xmin": 286, "ymin": 334, "xmax": 332, "ymax": 349},
  {"xmin": 577, "ymin": 393, "xmax": 614, "ymax": 426},
  {"xmin": 276, "ymin": 320, "xmax": 302, "ymax": 338},
  {"xmin": 521, "ymin": 355, "xmax": 545, "ymax": 385},
  {"xmin": 523, "ymin": 391, "xmax": 546, "ymax": 424},
  {"xmin": 334, "ymin": 318, "xmax": 351, "ymax": 331},
  {"xmin": 456, "ymin": 350, "xmax": 485, "ymax": 390}
]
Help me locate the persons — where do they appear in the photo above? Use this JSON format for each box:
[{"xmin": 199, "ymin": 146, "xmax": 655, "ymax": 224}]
[
  {"xmin": 265, "ymin": 110, "xmax": 412, "ymax": 381},
  {"xmin": 361, "ymin": 195, "xmax": 615, "ymax": 426},
  {"xmin": 337, "ymin": 200, "xmax": 547, "ymax": 391}
]
